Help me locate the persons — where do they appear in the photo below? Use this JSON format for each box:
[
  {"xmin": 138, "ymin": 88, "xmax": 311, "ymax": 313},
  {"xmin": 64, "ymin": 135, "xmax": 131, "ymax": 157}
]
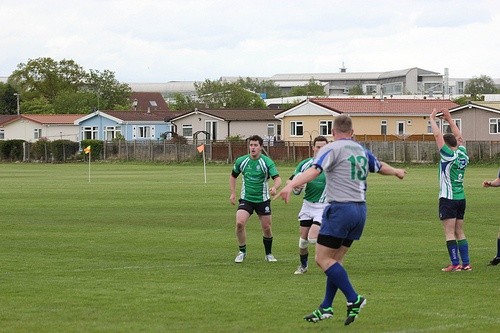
[
  {"xmin": 429, "ymin": 108, "xmax": 473, "ymax": 271},
  {"xmin": 483, "ymin": 170, "xmax": 500, "ymax": 266},
  {"xmin": 230, "ymin": 135, "xmax": 282, "ymax": 262},
  {"xmin": 287, "ymin": 136, "xmax": 327, "ymax": 274},
  {"xmin": 272, "ymin": 115, "xmax": 409, "ymax": 325}
]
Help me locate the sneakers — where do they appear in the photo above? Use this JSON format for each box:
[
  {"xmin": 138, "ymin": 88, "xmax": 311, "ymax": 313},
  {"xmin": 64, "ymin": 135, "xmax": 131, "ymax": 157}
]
[
  {"xmin": 303, "ymin": 306, "xmax": 334, "ymax": 322},
  {"xmin": 345, "ymin": 295, "xmax": 367, "ymax": 327},
  {"xmin": 265, "ymin": 253, "xmax": 277, "ymax": 261},
  {"xmin": 442, "ymin": 264, "xmax": 462, "ymax": 271},
  {"xmin": 461, "ymin": 264, "xmax": 472, "ymax": 270},
  {"xmin": 294, "ymin": 264, "xmax": 309, "ymax": 275},
  {"xmin": 235, "ymin": 252, "xmax": 247, "ymax": 262},
  {"xmin": 487, "ymin": 255, "xmax": 500, "ymax": 266}
]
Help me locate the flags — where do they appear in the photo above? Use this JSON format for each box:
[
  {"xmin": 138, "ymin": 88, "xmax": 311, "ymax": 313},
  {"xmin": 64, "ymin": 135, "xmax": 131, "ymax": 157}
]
[
  {"xmin": 84, "ymin": 146, "xmax": 91, "ymax": 154},
  {"xmin": 197, "ymin": 145, "xmax": 204, "ymax": 152}
]
